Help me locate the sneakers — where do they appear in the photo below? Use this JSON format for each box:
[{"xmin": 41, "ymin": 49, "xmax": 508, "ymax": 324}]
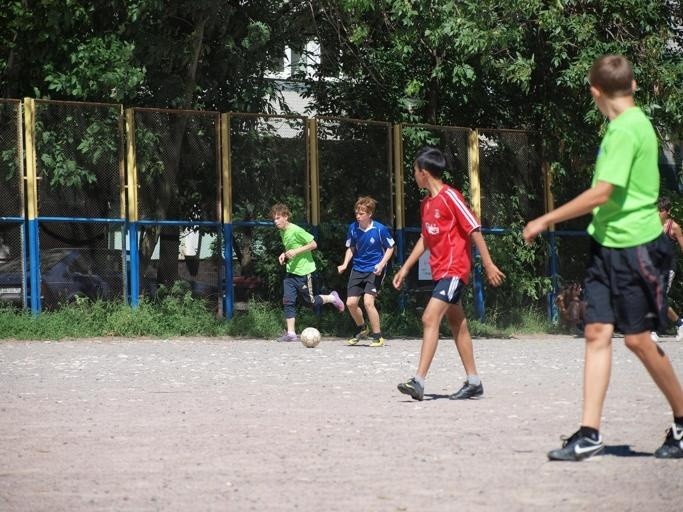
[
  {"xmin": 650, "ymin": 332, "xmax": 660, "ymax": 342},
  {"xmin": 655, "ymin": 422, "xmax": 682, "ymax": 457},
  {"xmin": 675, "ymin": 318, "xmax": 683, "ymax": 341},
  {"xmin": 348, "ymin": 324, "xmax": 369, "ymax": 345},
  {"xmin": 277, "ymin": 335, "xmax": 297, "ymax": 343},
  {"xmin": 397, "ymin": 377, "xmax": 424, "ymax": 401},
  {"xmin": 450, "ymin": 381, "xmax": 483, "ymax": 399},
  {"xmin": 547, "ymin": 428, "xmax": 605, "ymax": 461},
  {"xmin": 330, "ymin": 290, "xmax": 345, "ymax": 311},
  {"xmin": 369, "ymin": 336, "xmax": 384, "ymax": 347}
]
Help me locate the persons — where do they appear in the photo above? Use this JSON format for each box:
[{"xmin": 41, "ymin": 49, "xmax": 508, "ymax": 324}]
[
  {"xmin": 557, "ymin": 282, "xmax": 585, "ymax": 326},
  {"xmin": 337, "ymin": 195, "xmax": 396, "ymax": 348},
  {"xmin": 650, "ymin": 197, "xmax": 682, "ymax": 335},
  {"xmin": 523, "ymin": 54, "xmax": 682, "ymax": 461},
  {"xmin": 269, "ymin": 203, "xmax": 345, "ymax": 343},
  {"xmin": 392, "ymin": 148, "xmax": 507, "ymax": 401}
]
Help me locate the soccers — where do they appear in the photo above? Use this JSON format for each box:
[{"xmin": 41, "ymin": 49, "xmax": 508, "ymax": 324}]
[{"xmin": 300, "ymin": 326, "xmax": 321, "ymax": 348}]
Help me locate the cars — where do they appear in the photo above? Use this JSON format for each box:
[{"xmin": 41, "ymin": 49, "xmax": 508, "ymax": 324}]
[{"xmin": 0, "ymin": 242, "xmax": 226, "ymax": 317}]
